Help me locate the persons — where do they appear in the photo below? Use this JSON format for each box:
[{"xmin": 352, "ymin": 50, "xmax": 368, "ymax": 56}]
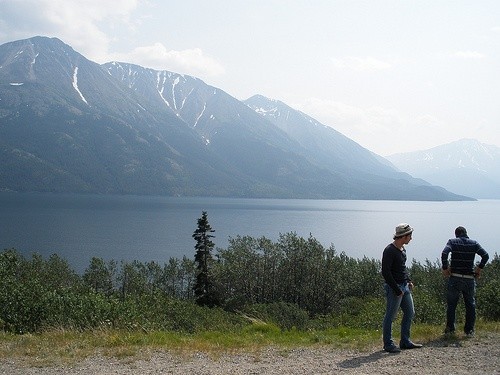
[
  {"xmin": 439, "ymin": 226, "xmax": 490, "ymax": 335},
  {"xmin": 379, "ymin": 223, "xmax": 423, "ymax": 352}
]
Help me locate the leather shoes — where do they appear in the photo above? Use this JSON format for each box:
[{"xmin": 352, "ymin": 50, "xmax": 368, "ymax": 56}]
[
  {"xmin": 384, "ymin": 343, "xmax": 402, "ymax": 352},
  {"xmin": 399, "ymin": 341, "xmax": 422, "ymax": 348}
]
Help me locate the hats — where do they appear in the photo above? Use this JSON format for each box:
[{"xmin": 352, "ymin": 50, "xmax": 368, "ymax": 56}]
[{"xmin": 393, "ymin": 223, "xmax": 414, "ymax": 236}]
